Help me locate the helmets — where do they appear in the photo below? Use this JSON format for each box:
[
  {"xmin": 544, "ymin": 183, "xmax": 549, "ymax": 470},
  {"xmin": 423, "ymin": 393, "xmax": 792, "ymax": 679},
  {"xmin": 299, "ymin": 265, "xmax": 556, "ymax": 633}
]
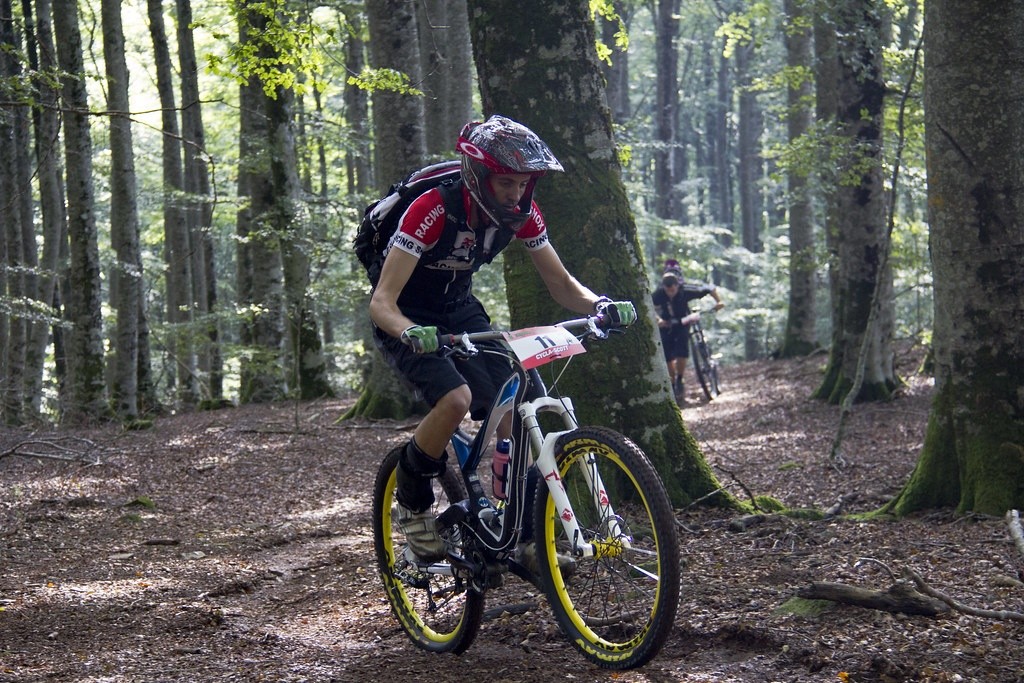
[{"xmin": 456, "ymin": 115, "xmax": 565, "ymax": 235}]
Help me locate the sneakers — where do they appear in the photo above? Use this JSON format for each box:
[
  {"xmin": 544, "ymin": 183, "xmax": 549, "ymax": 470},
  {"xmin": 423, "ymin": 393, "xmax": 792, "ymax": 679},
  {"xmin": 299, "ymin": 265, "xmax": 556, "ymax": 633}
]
[
  {"xmin": 396, "ymin": 490, "xmax": 445, "ymax": 561},
  {"xmin": 521, "ymin": 543, "xmax": 577, "ymax": 577}
]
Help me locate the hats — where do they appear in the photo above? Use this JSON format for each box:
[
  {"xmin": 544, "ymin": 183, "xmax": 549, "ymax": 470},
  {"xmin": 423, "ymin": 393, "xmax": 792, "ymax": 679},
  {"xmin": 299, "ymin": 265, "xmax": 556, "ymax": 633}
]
[{"xmin": 662, "ymin": 260, "xmax": 680, "ymax": 281}]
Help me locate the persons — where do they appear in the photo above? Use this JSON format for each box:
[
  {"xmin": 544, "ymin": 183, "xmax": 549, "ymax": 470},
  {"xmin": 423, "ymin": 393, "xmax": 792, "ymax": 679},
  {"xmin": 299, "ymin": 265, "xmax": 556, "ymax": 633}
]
[
  {"xmin": 364, "ymin": 112, "xmax": 640, "ymax": 584},
  {"xmin": 650, "ymin": 272, "xmax": 725, "ymax": 408}
]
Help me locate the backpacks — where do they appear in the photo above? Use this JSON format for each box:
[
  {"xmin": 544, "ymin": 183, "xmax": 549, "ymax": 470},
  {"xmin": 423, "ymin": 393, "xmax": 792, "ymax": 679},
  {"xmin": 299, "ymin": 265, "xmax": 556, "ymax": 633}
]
[{"xmin": 352, "ymin": 160, "xmax": 515, "ymax": 290}]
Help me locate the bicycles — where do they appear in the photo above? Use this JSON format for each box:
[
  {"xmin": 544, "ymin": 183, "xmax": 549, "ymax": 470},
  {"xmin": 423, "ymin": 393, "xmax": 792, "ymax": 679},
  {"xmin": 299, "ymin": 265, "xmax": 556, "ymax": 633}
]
[
  {"xmin": 666, "ymin": 305, "xmax": 724, "ymax": 401},
  {"xmin": 369, "ymin": 316, "xmax": 680, "ymax": 675}
]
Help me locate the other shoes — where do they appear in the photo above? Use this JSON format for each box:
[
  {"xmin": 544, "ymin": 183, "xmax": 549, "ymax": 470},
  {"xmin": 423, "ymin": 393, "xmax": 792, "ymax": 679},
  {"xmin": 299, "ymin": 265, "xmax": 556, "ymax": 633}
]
[{"xmin": 677, "ymin": 378, "xmax": 684, "ymax": 391}]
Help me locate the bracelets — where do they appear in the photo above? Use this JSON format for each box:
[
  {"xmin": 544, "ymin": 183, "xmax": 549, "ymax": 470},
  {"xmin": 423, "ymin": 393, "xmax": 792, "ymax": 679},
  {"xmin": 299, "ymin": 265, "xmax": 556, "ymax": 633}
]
[{"xmin": 593, "ymin": 294, "xmax": 615, "ymax": 314}]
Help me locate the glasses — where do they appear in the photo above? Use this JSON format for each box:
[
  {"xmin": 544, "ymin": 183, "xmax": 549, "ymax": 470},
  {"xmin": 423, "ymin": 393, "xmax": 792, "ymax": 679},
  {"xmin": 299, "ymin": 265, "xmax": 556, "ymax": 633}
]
[{"xmin": 664, "ymin": 277, "xmax": 680, "ymax": 287}]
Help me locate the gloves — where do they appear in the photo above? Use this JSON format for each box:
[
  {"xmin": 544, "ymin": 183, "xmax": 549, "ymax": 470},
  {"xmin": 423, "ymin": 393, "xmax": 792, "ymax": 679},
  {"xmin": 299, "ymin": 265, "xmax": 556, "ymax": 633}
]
[
  {"xmin": 401, "ymin": 325, "xmax": 444, "ymax": 354},
  {"xmin": 593, "ymin": 297, "xmax": 638, "ymax": 329}
]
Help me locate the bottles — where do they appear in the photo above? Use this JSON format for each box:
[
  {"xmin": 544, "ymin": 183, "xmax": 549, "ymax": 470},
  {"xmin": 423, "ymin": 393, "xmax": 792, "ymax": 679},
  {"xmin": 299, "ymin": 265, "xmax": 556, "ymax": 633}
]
[{"xmin": 491, "ymin": 439, "xmax": 512, "ymax": 501}]
